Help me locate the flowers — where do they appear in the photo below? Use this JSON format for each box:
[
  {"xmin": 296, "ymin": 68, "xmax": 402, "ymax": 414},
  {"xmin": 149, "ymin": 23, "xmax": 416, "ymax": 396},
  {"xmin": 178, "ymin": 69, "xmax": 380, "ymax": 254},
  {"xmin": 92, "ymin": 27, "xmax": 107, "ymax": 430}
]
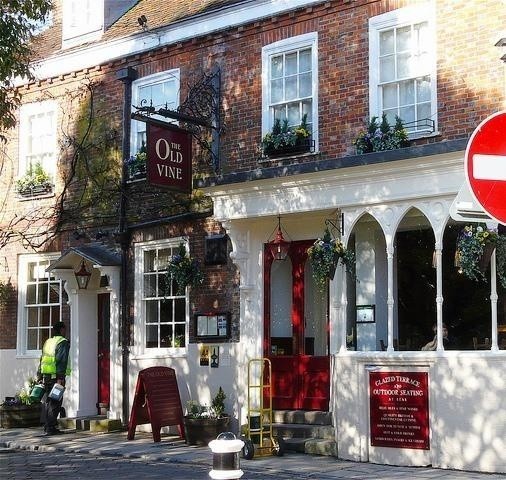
[
  {"xmin": 182, "ymin": 385, "xmax": 231, "ymax": 419},
  {"xmin": 262, "ymin": 112, "xmax": 313, "ymax": 150},
  {"xmin": 455, "ymin": 223, "xmax": 506, "ymax": 290},
  {"xmin": 306, "ymin": 227, "xmax": 360, "ymax": 297},
  {"xmin": 0, "ymin": 376, "xmax": 38, "ymax": 407},
  {"xmin": 159, "ymin": 253, "xmax": 207, "ymax": 303},
  {"xmin": 125, "ymin": 152, "xmax": 147, "ymax": 176},
  {"xmin": 351, "ymin": 110, "xmax": 411, "ymax": 154}
]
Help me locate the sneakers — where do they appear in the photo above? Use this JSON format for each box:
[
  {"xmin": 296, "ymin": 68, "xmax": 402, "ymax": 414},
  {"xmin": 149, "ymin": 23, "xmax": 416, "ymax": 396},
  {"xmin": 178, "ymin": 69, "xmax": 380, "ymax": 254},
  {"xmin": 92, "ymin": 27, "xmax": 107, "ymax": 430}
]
[{"xmin": 44, "ymin": 425, "xmax": 64, "ymax": 435}]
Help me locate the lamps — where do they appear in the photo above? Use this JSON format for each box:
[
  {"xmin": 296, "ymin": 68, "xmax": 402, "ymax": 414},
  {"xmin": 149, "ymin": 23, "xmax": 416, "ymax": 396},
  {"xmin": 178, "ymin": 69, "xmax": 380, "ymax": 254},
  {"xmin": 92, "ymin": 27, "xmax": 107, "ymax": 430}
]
[
  {"xmin": 267, "ymin": 216, "xmax": 294, "ymax": 263},
  {"xmin": 73, "ymin": 258, "xmax": 93, "ymax": 291}
]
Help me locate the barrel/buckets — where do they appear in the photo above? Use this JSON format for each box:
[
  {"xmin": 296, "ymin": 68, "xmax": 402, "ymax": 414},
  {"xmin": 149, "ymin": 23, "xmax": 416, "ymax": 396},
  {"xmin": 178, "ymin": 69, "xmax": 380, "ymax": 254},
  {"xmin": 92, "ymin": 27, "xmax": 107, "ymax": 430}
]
[
  {"xmin": 49, "ymin": 383, "xmax": 66, "ymax": 402},
  {"xmin": 30, "ymin": 379, "xmax": 47, "ymax": 401}
]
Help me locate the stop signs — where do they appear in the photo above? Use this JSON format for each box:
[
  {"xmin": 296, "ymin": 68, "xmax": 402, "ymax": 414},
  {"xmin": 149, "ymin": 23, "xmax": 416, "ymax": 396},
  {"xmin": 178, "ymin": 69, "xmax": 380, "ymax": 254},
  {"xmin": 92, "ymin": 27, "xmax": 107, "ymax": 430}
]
[{"xmin": 464, "ymin": 112, "xmax": 506, "ymax": 230}]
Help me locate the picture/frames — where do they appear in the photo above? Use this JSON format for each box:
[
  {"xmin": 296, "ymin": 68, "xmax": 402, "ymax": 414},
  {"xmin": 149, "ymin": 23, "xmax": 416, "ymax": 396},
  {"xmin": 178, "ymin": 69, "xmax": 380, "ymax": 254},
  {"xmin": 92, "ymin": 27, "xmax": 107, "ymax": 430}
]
[{"xmin": 356, "ymin": 305, "xmax": 376, "ymax": 322}]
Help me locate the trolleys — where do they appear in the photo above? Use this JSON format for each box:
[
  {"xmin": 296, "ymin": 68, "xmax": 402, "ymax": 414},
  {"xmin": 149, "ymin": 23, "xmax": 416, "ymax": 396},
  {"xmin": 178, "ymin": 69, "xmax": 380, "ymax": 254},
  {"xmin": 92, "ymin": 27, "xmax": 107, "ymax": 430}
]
[{"xmin": 239, "ymin": 357, "xmax": 286, "ymax": 460}]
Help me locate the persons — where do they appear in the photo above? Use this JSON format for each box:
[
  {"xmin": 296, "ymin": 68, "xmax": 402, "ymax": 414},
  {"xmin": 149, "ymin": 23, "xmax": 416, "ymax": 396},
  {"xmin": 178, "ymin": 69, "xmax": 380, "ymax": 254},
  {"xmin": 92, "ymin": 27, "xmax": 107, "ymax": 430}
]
[
  {"xmin": 34, "ymin": 321, "xmax": 71, "ymax": 437},
  {"xmin": 421, "ymin": 322, "xmax": 450, "ymax": 352}
]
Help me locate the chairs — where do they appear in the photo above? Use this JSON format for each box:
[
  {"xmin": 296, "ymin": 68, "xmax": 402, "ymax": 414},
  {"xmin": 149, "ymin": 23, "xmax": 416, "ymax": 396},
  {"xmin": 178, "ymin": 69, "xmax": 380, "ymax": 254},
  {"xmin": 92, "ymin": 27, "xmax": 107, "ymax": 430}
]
[{"xmin": 380, "ymin": 337, "xmax": 491, "ymax": 350}]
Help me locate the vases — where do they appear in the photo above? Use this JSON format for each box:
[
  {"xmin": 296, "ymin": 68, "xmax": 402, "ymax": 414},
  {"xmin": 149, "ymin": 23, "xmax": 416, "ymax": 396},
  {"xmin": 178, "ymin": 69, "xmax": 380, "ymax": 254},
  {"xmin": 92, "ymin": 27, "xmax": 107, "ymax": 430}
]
[
  {"xmin": 0, "ymin": 408, "xmax": 43, "ymax": 429},
  {"xmin": 479, "ymin": 244, "xmax": 495, "ymax": 274},
  {"xmin": 184, "ymin": 421, "xmax": 231, "ymax": 445},
  {"xmin": 327, "ymin": 253, "xmax": 340, "ymax": 281}
]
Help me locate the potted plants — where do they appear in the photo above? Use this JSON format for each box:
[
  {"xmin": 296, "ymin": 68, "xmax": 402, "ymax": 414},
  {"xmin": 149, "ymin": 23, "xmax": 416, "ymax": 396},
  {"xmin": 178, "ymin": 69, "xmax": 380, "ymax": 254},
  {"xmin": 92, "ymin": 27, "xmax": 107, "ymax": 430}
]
[{"xmin": 13, "ymin": 162, "xmax": 55, "ymax": 197}]
